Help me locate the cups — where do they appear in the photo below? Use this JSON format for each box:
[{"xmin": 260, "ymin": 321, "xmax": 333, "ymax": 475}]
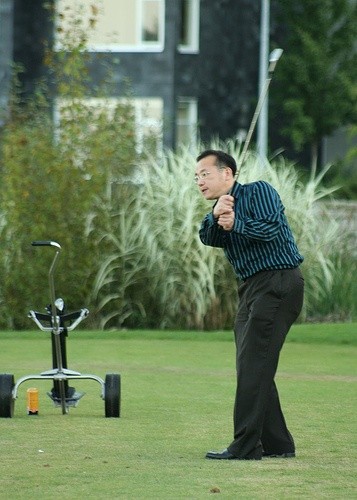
[{"xmin": 28, "ymin": 388, "xmax": 39, "ymax": 417}]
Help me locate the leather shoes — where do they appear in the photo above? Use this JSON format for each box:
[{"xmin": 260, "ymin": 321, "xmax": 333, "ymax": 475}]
[
  {"xmin": 206, "ymin": 448, "xmax": 263, "ymax": 460},
  {"xmin": 262, "ymin": 449, "xmax": 295, "ymax": 458}
]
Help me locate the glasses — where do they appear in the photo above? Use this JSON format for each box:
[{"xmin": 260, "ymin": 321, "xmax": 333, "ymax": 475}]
[{"xmin": 194, "ymin": 169, "xmax": 227, "ymax": 183}]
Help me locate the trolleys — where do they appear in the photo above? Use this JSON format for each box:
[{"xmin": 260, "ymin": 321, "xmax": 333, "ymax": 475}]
[{"xmin": 1, "ymin": 240, "xmax": 122, "ymax": 418}]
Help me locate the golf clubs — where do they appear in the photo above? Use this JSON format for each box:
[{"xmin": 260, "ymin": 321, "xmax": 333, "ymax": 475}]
[{"xmin": 231, "ymin": 47, "xmax": 284, "ymax": 198}]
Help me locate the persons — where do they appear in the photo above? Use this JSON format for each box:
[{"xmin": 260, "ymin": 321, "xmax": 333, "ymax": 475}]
[{"xmin": 195, "ymin": 149, "xmax": 305, "ymax": 460}]
